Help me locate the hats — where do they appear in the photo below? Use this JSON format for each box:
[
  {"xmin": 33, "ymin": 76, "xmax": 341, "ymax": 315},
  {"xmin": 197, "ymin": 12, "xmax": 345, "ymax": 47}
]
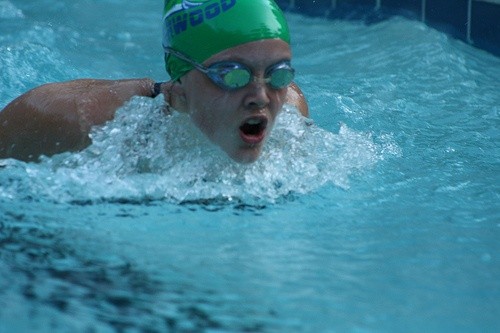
[{"xmin": 163, "ymin": 0, "xmax": 292, "ymax": 84}]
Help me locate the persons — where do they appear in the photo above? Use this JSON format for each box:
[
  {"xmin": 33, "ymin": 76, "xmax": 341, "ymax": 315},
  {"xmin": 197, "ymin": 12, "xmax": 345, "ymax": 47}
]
[{"xmin": 0, "ymin": 0, "xmax": 309, "ymax": 166}]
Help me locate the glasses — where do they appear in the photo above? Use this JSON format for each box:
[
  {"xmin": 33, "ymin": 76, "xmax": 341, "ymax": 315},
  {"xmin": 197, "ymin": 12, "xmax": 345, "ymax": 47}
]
[{"xmin": 164, "ymin": 46, "xmax": 297, "ymax": 90}]
